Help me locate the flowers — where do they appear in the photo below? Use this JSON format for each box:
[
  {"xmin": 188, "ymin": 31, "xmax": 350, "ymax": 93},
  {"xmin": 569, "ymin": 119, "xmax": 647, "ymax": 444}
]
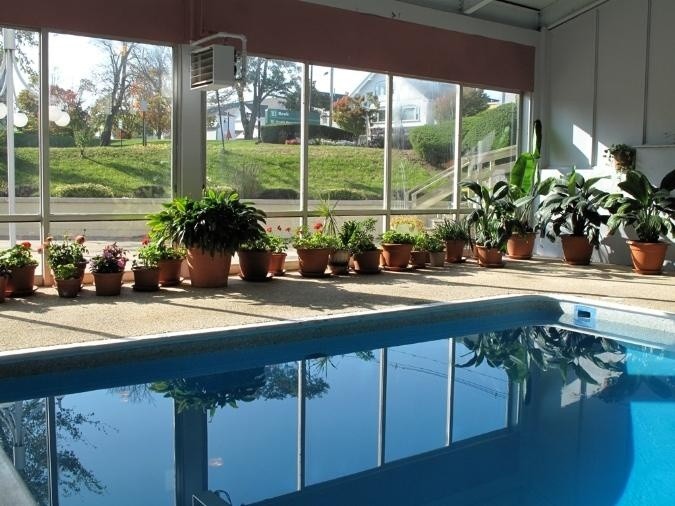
[
  {"xmin": 89, "ymin": 241, "xmax": 128, "ymax": 272},
  {"xmin": 0, "ymin": 242, "xmax": 36, "ymax": 267},
  {"xmin": 132, "ymin": 236, "xmax": 161, "ymax": 269},
  {"xmin": 264, "ymin": 225, "xmax": 291, "ymax": 254},
  {"xmin": 291, "ymin": 223, "xmax": 334, "ymax": 249},
  {"xmin": 37, "ymin": 229, "xmax": 89, "ymax": 264}
]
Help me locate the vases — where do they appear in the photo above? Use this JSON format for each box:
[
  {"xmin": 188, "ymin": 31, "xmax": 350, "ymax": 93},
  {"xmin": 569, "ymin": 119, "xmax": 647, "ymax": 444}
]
[
  {"xmin": 268, "ymin": 253, "xmax": 286, "ymax": 276},
  {"xmin": 296, "ymin": 245, "xmax": 334, "ymax": 278},
  {"xmin": 92, "ymin": 271, "xmax": 124, "ymax": 295},
  {"xmin": 4, "ymin": 263, "xmax": 38, "ymax": 297},
  {"xmin": 132, "ymin": 265, "xmax": 163, "ymax": 291},
  {"xmin": 51, "ymin": 260, "xmax": 88, "ymax": 291}
]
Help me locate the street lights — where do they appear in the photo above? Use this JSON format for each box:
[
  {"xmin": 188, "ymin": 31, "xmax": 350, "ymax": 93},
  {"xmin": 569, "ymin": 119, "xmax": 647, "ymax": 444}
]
[{"xmin": 0, "ymin": 27, "xmax": 71, "ymax": 248}]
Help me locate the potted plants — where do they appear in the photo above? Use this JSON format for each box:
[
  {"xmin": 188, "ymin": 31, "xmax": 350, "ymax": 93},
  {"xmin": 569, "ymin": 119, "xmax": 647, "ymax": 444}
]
[
  {"xmin": 154, "ymin": 244, "xmax": 186, "ymax": 286},
  {"xmin": 0, "ymin": 264, "xmax": 15, "ymax": 302},
  {"xmin": 144, "ymin": 187, "xmax": 268, "ymax": 288},
  {"xmin": 53, "ymin": 264, "xmax": 81, "ymax": 298},
  {"xmin": 234, "ymin": 234, "xmax": 272, "ymax": 283}
]
